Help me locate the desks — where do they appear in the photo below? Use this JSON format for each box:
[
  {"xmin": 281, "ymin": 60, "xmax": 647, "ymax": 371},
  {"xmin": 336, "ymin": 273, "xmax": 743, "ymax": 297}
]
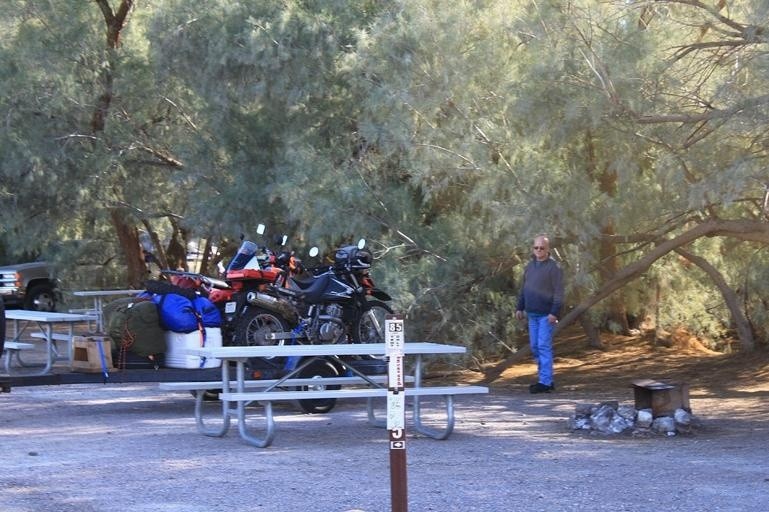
[
  {"xmin": 174, "ymin": 341, "xmax": 468, "ymax": 447},
  {"xmin": 4, "ymin": 309, "xmax": 98, "ymax": 374},
  {"xmin": 73, "ymin": 290, "xmax": 147, "ymax": 336}
]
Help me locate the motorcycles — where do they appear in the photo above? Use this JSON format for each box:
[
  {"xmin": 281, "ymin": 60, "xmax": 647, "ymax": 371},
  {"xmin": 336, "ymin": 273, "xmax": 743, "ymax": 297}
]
[{"xmin": 166, "ymin": 223, "xmax": 395, "ymax": 371}]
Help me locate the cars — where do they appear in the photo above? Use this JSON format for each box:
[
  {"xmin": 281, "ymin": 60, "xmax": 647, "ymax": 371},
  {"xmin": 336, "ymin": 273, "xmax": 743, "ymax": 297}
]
[{"xmin": 1, "ymin": 237, "xmax": 161, "ymax": 312}]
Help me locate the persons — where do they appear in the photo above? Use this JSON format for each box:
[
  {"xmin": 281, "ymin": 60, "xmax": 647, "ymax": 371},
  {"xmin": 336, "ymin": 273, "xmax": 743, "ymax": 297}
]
[{"xmin": 512, "ymin": 234, "xmax": 565, "ymax": 393}]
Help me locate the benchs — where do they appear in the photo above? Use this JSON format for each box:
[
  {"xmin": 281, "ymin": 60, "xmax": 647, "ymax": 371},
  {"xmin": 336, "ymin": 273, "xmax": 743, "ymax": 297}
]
[
  {"xmin": 218, "ymin": 385, "xmax": 490, "ymax": 402},
  {"xmin": 68, "ymin": 308, "xmax": 98, "ymax": 314},
  {"xmin": 4, "ymin": 342, "xmax": 33, "ymax": 350},
  {"xmin": 30, "ymin": 332, "xmax": 77, "ymax": 341},
  {"xmin": 159, "ymin": 373, "xmax": 415, "ymax": 390},
  {"xmin": 86, "ymin": 311, "xmax": 104, "ymax": 315}
]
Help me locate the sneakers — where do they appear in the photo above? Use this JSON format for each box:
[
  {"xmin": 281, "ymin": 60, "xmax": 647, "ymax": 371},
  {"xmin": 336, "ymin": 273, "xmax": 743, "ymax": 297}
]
[{"xmin": 529, "ymin": 381, "xmax": 555, "ymax": 394}]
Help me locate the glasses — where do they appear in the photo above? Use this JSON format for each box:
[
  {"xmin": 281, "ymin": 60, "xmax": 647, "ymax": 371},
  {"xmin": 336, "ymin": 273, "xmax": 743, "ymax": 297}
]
[{"xmin": 533, "ymin": 246, "xmax": 546, "ymax": 250}]
